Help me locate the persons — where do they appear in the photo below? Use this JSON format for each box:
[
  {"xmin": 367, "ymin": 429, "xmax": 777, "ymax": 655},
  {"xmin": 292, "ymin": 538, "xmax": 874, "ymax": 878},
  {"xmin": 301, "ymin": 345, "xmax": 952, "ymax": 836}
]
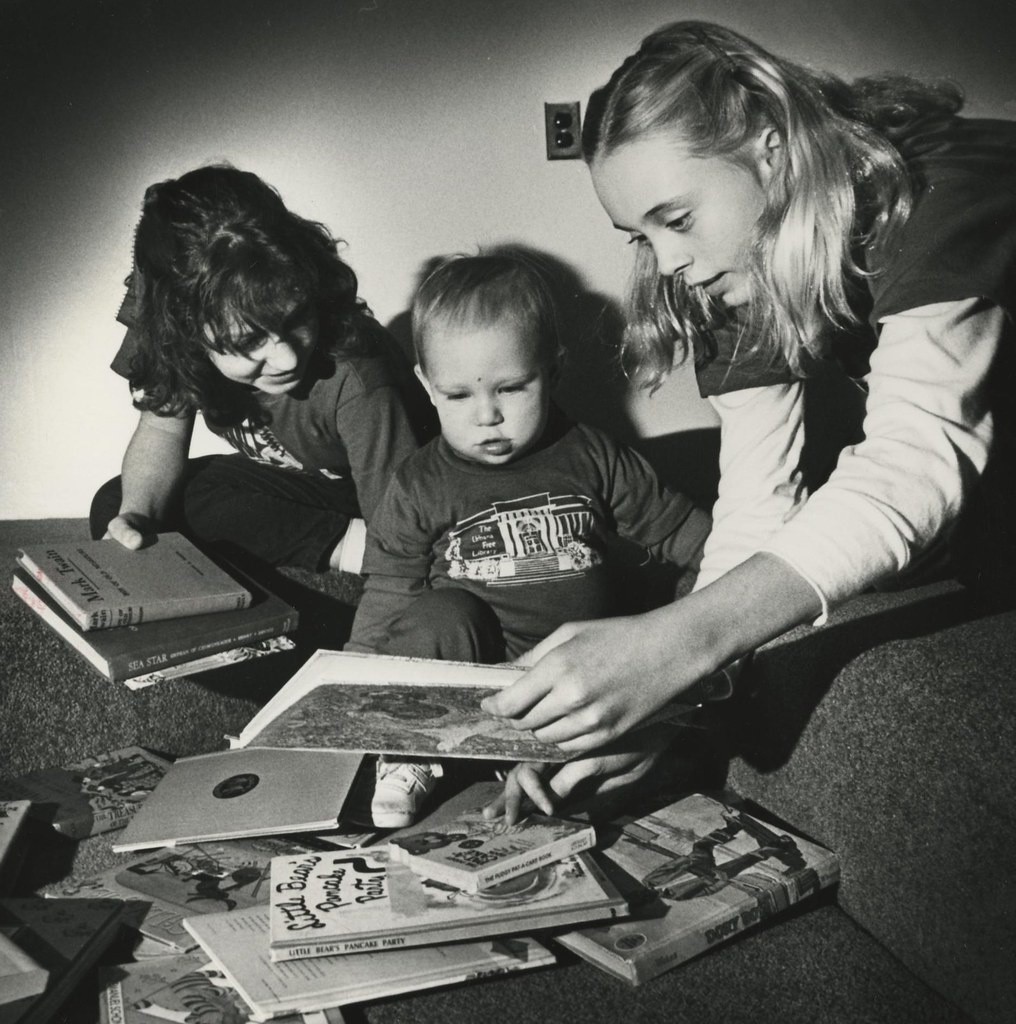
[
  {"xmin": 483, "ymin": 20, "xmax": 1016, "ymax": 828},
  {"xmin": 89, "ymin": 162, "xmax": 426, "ymax": 665},
  {"xmin": 344, "ymin": 256, "xmax": 713, "ymax": 830}
]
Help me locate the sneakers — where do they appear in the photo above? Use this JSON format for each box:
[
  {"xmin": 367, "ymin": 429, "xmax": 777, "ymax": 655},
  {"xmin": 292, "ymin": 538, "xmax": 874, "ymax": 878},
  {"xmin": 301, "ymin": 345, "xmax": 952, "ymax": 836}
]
[{"xmin": 371, "ymin": 756, "xmax": 444, "ymax": 828}]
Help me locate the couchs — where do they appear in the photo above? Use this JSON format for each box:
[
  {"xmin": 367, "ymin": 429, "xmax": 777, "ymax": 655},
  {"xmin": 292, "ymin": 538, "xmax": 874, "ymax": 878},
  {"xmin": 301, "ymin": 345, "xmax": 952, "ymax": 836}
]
[{"xmin": 0, "ymin": 565, "xmax": 1015, "ymax": 1024}]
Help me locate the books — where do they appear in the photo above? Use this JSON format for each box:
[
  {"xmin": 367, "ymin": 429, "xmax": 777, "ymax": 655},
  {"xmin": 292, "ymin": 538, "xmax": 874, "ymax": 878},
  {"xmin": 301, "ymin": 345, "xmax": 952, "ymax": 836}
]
[{"xmin": 0, "ymin": 533, "xmax": 840, "ymax": 1024}]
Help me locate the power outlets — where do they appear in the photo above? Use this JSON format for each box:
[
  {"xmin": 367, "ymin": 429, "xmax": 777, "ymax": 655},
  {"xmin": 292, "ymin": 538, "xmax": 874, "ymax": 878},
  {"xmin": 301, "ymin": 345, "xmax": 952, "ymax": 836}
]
[{"xmin": 544, "ymin": 101, "xmax": 581, "ymax": 160}]
